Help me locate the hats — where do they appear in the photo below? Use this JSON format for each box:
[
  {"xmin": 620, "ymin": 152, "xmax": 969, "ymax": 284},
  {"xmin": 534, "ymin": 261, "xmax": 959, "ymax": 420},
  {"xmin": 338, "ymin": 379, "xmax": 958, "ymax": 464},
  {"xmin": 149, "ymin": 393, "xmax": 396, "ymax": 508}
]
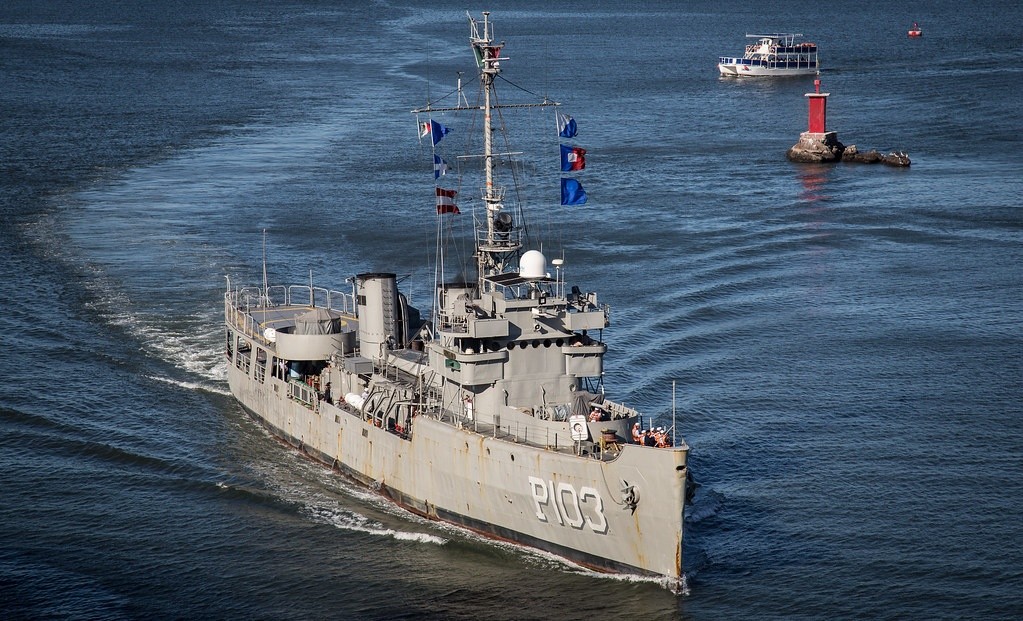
[
  {"xmin": 651, "ymin": 427, "xmax": 654, "ymax": 431},
  {"xmin": 660, "ymin": 430, "xmax": 664, "ymax": 433},
  {"xmin": 641, "ymin": 430, "xmax": 646, "ymax": 433},
  {"xmin": 657, "ymin": 427, "xmax": 661, "ymax": 430},
  {"xmin": 635, "ymin": 422, "xmax": 639, "ymax": 426},
  {"xmin": 595, "ymin": 408, "xmax": 599, "ymax": 410}
]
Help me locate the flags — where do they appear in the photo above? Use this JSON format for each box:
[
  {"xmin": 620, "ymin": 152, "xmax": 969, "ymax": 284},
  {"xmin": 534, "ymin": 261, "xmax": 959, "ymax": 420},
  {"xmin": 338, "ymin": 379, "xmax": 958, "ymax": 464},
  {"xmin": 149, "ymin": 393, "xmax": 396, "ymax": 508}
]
[
  {"xmin": 556, "ymin": 111, "xmax": 579, "ymax": 138},
  {"xmin": 560, "ymin": 177, "xmax": 589, "ymax": 205},
  {"xmin": 559, "ymin": 144, "xmax": 586, "ymax": 172},
  {"xmin": 434, "ymin": 187, "xmax": 462, "ymax": 215}
]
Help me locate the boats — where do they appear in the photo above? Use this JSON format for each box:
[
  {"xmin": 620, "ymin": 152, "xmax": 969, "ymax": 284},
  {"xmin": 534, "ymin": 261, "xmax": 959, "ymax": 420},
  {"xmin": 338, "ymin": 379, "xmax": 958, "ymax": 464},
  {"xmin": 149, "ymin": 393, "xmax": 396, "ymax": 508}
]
[
  {"xmin": 908, "ymin": 21, "xmax": 922, "ymax": 36},
  {"xmin": 224, "ymin": 11, "xmax": 701, "ymax": 577},
  {"xmin": 718, "ymin": 32, "xmax": 820, "ymax": 76}
]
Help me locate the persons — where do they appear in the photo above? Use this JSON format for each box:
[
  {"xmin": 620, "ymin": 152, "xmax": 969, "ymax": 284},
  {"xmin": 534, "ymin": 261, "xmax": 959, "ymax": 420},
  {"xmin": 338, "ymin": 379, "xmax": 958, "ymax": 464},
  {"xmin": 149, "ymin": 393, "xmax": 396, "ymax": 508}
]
[
  {"xmin": 588, "ymin": 407, "xmax": 606, "ymax": 423},
  {"xmin": 307, "ymin": 374, "xmax": 336, "ymax": 407},
  {"xmin": 366, "ymin": 408, "xmax": 408, "ymax": 442},
  {"xmin": 633, "ymin": 423, "xmax": 671, "ymax": 449}
]
